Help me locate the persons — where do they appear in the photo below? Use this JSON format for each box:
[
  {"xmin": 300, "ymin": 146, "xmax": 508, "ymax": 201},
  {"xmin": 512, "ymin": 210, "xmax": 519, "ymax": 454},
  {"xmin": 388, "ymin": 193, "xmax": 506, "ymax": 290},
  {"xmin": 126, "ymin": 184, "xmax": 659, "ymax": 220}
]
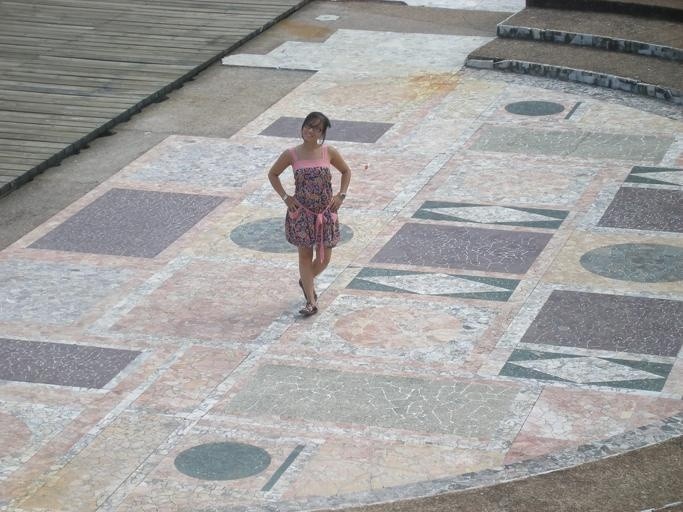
[{"xmin": 268, "ymin": 112, "xmax": 352, "ymax": 314}]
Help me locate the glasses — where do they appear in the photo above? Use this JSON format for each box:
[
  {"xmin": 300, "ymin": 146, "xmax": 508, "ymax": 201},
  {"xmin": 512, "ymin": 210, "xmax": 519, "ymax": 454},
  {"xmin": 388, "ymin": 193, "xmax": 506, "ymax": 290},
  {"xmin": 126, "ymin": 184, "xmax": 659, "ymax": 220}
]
[{"xmin": 304, "ymin": 123, "xmax": 321, "ymax": 133}]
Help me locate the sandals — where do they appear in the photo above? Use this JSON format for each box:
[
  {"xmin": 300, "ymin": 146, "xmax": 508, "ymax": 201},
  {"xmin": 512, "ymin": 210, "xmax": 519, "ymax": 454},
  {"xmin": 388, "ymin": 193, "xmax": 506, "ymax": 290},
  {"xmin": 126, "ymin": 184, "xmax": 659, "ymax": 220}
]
[
  {"xmin": 299, "ymin": 279, "xmax": 318, "ymax": 302},
  {"xmin": 299, "ymin": 303, "xmax": 318, "ymax": 315}
]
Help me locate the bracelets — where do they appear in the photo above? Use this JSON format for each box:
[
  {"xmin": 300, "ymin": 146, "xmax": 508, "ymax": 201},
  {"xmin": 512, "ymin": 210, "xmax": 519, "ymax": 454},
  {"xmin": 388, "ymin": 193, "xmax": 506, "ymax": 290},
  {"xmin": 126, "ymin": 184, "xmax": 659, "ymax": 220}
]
[
  {"xmin": 282, "ymin": 194, "xmax": 288, "ymax": 201},
  {"xmin": 338, "ymin": 192, "xmax": 346, "ymax": 199}
]
